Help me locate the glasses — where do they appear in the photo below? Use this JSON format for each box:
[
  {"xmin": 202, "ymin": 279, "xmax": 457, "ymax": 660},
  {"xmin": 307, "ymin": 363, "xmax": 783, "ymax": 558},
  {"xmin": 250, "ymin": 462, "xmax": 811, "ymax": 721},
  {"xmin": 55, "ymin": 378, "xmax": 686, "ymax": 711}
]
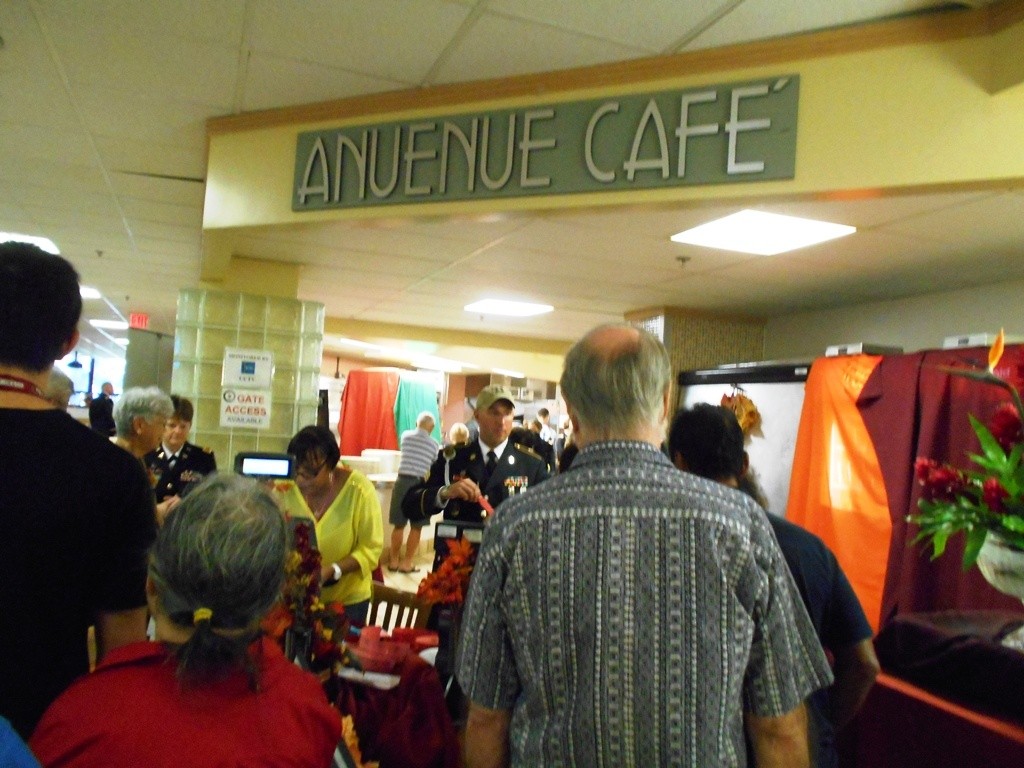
[{"xmin": 293, "ymin": 457, "xmax": 328, "ymax": 479}]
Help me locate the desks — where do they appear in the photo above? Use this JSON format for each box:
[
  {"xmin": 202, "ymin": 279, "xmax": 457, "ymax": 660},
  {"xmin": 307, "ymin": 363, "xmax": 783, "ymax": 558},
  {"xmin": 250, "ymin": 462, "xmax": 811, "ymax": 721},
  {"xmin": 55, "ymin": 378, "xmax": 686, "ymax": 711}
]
[
  {"xmin": 861, "ymin": 611, "xmax": 1024, "ymax": 768},
  {"xmin": 339, "ymin": 619, "xmax": 462, "ymax": 768}
]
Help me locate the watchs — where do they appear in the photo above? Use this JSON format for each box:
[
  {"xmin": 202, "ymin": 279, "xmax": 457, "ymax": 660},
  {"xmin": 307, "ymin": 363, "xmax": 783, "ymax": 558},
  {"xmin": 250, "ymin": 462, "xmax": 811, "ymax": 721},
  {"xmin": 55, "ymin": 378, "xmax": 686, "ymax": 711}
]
[{"xmin": 332, "ymin": 563, "xmax": 342, "ymax": 580}]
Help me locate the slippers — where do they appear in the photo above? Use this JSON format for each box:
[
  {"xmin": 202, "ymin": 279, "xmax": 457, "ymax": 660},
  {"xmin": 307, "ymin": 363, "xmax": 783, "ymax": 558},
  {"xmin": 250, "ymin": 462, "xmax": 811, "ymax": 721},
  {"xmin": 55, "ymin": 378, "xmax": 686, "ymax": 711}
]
[{"xmin": 387, "ymin": 559, "xmax": 420, "ymax": 572}]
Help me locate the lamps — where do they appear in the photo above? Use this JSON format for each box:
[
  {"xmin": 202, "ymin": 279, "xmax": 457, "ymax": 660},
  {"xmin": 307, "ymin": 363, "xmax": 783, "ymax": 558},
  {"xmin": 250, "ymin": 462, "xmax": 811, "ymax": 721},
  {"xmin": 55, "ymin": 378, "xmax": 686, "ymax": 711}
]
[{"xmin": 68, "ymin": 351, "xmax": 82, "ymax": 368}]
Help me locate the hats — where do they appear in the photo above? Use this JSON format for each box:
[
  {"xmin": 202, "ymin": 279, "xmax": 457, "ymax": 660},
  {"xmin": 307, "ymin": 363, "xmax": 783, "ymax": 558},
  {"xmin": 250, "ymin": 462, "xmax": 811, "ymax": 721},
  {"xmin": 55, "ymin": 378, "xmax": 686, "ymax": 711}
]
[
  {"xmin": 476, "ymin": 385, "xmax": 516, "ymax": 411},
  {"xmin": 538, "ymin": 408, "xmax": 549, "ymax": 418}
]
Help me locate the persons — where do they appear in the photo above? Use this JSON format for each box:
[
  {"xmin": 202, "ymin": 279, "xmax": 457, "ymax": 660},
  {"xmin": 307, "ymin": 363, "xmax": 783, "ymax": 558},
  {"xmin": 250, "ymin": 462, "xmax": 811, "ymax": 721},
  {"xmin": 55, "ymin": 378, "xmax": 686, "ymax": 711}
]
[
  {"xmin": 455, "ymin": 322, "xmax": 834, "ymax": 768},
  {"xmin": 29, "ymin": 469, "xmax": 342, "ymax": 768},
  {"xmin": 42, "ymin": 369, "xmax": 579, "ymax": 698},
  {"xmin": 0, "ymin": 240, "xmax": 157, "ymax": 742},
  {"xmin": 668, "ymin": 404, "xmax": 880, "ymax": 768}
]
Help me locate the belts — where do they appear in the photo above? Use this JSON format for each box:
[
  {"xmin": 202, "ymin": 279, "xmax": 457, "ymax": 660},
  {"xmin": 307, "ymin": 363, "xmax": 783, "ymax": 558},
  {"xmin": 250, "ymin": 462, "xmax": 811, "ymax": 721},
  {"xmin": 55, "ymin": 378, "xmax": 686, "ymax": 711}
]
[{"xmin": 398, "ymin": 473, "xmax": 425, "ymax": 481}]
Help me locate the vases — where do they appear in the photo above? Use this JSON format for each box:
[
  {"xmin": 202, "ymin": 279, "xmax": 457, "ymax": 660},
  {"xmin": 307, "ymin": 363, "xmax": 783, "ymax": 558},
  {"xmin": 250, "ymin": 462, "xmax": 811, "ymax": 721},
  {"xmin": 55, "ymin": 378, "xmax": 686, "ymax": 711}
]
[{"xmin": 977, "ymin": 528, "xmax": 1024, "ymax": 653}]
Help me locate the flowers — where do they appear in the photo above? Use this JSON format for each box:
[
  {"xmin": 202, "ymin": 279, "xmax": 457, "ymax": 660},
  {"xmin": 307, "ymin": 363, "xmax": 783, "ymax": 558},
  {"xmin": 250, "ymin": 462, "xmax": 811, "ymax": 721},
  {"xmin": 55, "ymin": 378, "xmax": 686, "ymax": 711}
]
[
  {"xmin": 413, "ymin": 536, "xmax": 474, "ymax": 613},
  {"xmin": 279, "ymin": 523, "xmax": 353, "ymax": 670},
  {"xmin": 903, "ymin": 327, "xmax": 1024, "ymax": 574}
]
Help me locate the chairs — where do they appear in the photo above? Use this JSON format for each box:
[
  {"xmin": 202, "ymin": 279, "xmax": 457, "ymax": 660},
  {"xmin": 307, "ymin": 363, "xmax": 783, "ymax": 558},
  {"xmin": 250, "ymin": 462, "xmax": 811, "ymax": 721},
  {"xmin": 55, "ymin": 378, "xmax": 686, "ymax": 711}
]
[{"xmin": 369, "ymin": 583, "xmax": 432, "ymax": 629}]
